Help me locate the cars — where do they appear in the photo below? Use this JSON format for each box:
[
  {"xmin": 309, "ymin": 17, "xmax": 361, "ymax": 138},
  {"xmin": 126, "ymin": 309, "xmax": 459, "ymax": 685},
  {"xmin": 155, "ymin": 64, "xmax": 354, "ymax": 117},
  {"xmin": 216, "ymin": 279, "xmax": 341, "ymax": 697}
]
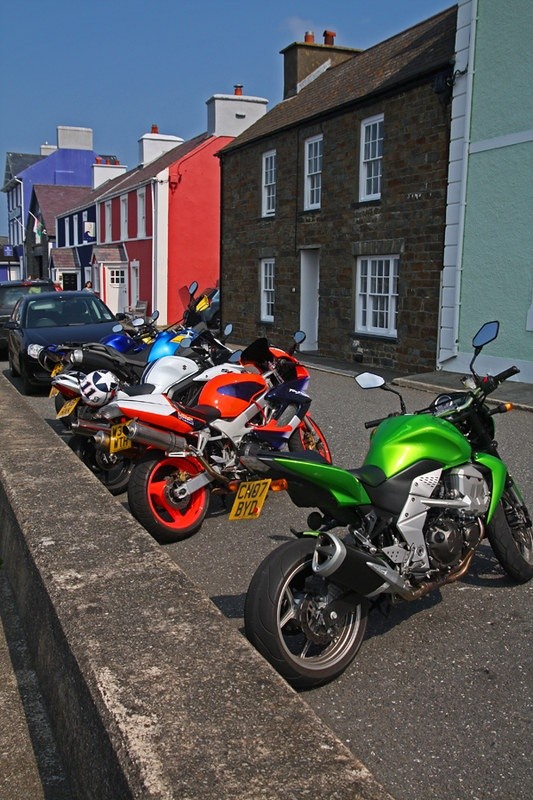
[{"xmin": 3, "ymin": 291, "xmax": 127, "ymax": 395}]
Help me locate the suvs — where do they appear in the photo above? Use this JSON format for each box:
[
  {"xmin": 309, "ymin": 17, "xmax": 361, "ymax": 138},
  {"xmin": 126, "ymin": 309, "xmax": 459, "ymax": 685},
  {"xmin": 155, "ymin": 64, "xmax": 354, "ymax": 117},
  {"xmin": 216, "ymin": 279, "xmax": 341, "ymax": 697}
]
[{"xmin": 0, "ymin": 276, "xmax": 63, "ymax": 355}]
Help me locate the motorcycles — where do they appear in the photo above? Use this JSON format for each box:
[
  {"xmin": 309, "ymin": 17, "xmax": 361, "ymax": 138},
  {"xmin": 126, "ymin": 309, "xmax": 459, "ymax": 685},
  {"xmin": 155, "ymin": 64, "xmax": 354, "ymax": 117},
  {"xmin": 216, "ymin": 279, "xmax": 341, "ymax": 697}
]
[
  {"xmin": 227, "ymin": 322, "xmax": 533, "ymax": 687},
  {"xmin": 97, "ymin": 332, "xmax": 333, "ymax": 545},
  {"xmin": 37, "ymin": 282, "xmax": 249, "ymax": 495}
]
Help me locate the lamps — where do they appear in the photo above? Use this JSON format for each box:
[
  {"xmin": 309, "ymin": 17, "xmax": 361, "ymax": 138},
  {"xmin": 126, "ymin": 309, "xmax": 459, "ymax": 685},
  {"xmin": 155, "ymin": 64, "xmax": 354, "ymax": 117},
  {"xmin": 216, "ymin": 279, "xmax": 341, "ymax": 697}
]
[{"xmin": 446, "ymin": 67, "xmax": 467, "ymax": 87}]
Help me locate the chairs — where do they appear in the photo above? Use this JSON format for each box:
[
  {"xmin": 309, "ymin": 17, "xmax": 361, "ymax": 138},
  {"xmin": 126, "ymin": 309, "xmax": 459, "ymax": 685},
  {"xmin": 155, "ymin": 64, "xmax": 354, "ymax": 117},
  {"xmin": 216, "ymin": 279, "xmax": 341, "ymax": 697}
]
[{"xmin": 61, "ymin": 303, "xmax": 85, "ymax": 325}]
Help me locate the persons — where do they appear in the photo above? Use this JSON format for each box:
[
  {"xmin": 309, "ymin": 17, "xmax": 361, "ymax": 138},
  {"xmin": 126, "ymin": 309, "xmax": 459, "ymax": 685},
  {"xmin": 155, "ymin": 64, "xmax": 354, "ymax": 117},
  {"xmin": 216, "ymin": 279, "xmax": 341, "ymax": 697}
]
[
  {"xmin": 25, "ymin": 275, "xmax": 41, "ymax": 294},
  {"xmin": 83, "ymin": 281, "xmax": 94, "ymax": 293}
]
